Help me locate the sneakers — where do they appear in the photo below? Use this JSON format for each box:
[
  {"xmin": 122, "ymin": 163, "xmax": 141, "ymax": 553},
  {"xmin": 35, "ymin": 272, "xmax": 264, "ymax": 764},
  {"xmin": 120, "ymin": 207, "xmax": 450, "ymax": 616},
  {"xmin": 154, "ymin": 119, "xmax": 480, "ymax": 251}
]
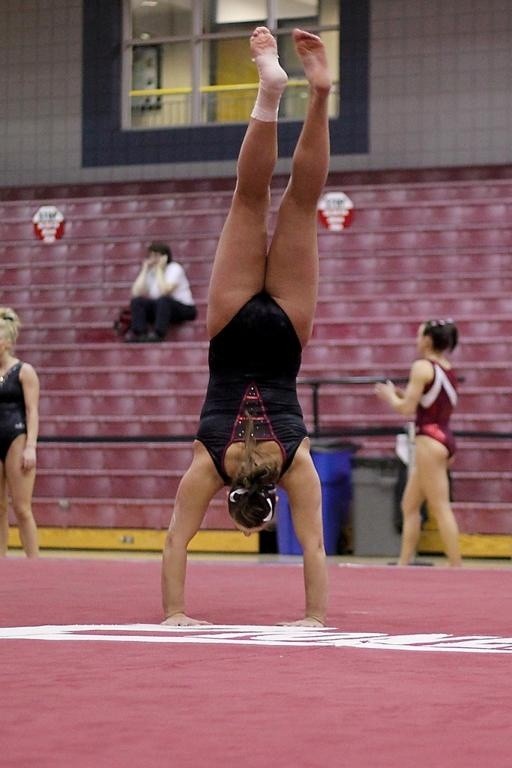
[{"xmin": 125, "ymin": 328, "xmax": 162, "ymax": 341}]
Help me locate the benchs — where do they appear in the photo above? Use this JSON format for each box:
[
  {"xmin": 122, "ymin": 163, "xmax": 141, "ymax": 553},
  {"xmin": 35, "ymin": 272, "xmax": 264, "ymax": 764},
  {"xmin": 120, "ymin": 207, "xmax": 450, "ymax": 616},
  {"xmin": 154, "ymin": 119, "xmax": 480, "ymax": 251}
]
[{"xmin": 1, "ymin": 179, "xmax": 512, "ymax": 538}]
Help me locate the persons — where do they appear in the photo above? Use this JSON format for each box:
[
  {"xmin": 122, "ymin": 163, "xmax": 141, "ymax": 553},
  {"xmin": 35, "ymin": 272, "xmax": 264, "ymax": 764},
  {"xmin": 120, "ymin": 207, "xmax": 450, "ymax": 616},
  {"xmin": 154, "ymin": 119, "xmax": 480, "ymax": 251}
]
[
  {"xmin": 156, "ymin": 24, "xmax": 334, "ymax": 634},
  {"xmin": 374, "ymin": 317, "xmax": 465, "ymax": 568},
  {"xmin": 0, "ymin": 304, "xmax": 44, "ymax": 557},
  {"xmin": 120, "ymin": 245, "xmax": 199, "ymax": 345}
]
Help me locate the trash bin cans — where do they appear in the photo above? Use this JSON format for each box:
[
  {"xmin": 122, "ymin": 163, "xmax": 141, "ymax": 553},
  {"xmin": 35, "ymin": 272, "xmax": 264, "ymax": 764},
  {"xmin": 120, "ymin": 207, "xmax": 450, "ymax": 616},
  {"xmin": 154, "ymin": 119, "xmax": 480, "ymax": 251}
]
[
  {"xmin": 276, "ymin": 443, "xmax": 353, "ymax": 556},
  {"xmin": 350, "ymin": 457, "xmax": 423, "ymax": 558}
]
